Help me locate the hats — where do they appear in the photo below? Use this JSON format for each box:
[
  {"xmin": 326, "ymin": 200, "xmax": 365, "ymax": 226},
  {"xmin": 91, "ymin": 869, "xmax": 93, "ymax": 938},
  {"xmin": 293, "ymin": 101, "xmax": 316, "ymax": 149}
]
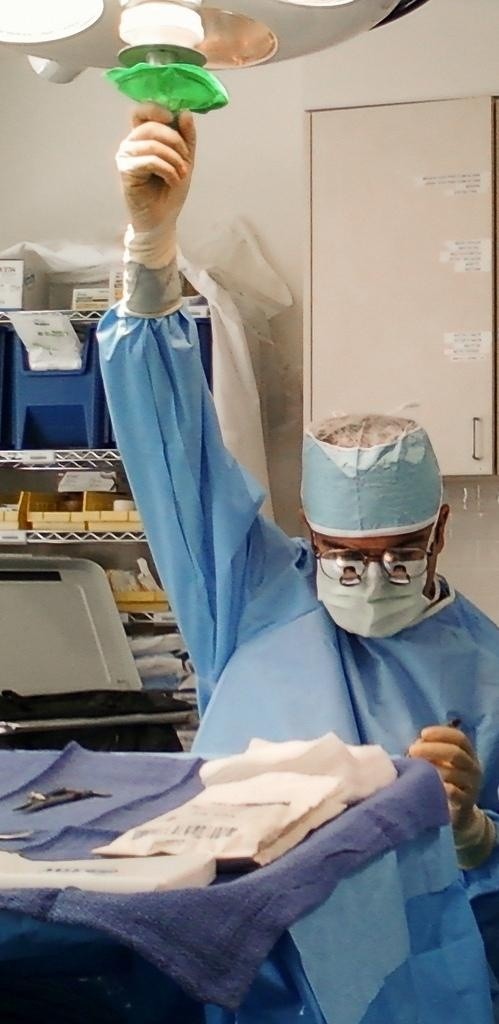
[{"xmin": 299, "ymin": 406, "xmax": 449, "ymax": 540}]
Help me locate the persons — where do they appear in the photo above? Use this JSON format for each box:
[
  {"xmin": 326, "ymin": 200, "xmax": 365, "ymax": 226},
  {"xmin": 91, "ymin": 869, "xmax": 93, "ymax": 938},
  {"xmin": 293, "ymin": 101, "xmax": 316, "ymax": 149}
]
[{"xmin": 96, "ymin": 101, "xmax": 499, "ymax": 900}]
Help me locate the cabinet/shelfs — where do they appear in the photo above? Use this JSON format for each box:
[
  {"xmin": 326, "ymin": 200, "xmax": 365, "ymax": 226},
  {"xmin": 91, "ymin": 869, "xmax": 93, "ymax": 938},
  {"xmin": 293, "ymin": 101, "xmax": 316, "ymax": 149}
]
[
  {"xmin": 0, "ymin": 306, "xmax": 228, "ymax": 700},
  {"xmin": 305, "ymin": 94, "xmax": 498, "ymax": 487}
]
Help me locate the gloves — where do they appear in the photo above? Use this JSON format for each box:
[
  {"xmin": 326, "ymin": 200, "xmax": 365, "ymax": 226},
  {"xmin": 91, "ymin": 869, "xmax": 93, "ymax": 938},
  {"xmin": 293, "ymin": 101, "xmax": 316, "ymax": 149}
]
[
  {"xmin": 407, "ymin": 724, "xmax": 496, "ymax": 871},
  {"xmin": 114, "ymin": 99, "xmax": 198, "ymax": 320}
]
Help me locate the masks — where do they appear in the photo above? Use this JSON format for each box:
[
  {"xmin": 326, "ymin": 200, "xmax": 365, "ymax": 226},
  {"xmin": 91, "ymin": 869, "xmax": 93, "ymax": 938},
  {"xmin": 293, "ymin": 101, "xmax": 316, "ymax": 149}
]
[{"xmin": 314, "ymin": 508, "xmax": 443, "ymax": 640}]
